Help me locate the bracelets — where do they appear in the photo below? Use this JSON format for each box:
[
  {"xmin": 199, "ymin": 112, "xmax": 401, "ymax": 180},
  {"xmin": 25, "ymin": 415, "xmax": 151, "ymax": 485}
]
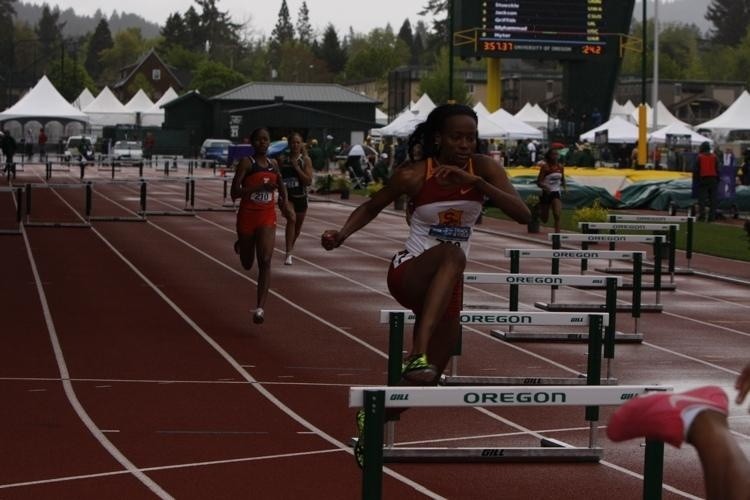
[{"xmin": 283, "ymin": 203, "xmax": 292, "ymax": 209}]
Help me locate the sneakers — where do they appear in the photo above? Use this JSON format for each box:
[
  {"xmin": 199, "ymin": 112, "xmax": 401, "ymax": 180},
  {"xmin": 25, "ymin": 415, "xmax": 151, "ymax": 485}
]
[
  {"xmin": 285, "ymin": 255, "xmax": 293, "ymax": 265},
  {"xmin": 253, "ymin": 308, "xmax": 264, "ymax": 323},
  {"xmin": 354, "ymin": 407, "xmax": 365, "ymax": 468},
  {"xmin": 606, "ymin": 384, "xmax": 729, "ymax": 449},
  {"xmin": 401, "ymin": 352, "xmax": 437, "ymax": 382}
]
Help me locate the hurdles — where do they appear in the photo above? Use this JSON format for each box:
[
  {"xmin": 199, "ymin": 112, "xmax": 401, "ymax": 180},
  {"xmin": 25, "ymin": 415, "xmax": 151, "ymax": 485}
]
[
  {"xmin": 492, "ymin": 249, "xmax": 647, "ymax": 346},
  {"xmin": 594, "ymin": 214, "xmax": 694, "ymax": 275},
  {"xmin": 578, "ymin": 221, "xmax": 680, "ymax": 292},
  {"xmin": 534, "ymin": 234, "xmax": 667, "ymax": 313},
  {"xmin": 377, "ymin": 308, "xmax": 611, "ymax": 466},
  {"xmin": 0, "ymin": 152, "xmax": 243, "ymax": 235},
  {"xmin": 347, "ymin": 385, "xmax": 676, "ymax": 498},
  {"xmin": 439, "ymin": 269, "xmax": 619, "ymax": 385}
]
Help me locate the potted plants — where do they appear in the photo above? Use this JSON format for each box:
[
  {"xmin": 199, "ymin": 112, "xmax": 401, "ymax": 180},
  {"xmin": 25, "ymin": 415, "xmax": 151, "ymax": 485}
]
[
  {"xmin": 337, "ymin": 176, "xmax": 352, "ymax": 199},
  {"xmin": 573, "ymin": 199, "xmax": 607, "ymax": 245},
  {"xmin": 525, "ymin": 195, "xmax": 542, "ymax": 232}
]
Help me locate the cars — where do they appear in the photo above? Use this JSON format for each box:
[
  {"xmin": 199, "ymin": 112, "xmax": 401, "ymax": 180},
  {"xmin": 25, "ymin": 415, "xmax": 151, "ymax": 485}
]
[
  {"xmin": 511, "ymin": 175, "xmax": 542, "ymax": 190},
  {"xmin": 64, "ymin": 136, "xmax": 95, "ymax": 166},
  {"xmin": 112, "ymin": 141, "xmax": 144, "ymax": 167},
  {"xmin": 200, "ymin": 138, "xmax": 232, "ymax": 168}
]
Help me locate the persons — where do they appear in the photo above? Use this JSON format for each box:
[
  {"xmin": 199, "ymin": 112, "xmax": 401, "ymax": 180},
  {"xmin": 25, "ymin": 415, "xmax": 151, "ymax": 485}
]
[
  {"xmin": 25, "ymin": 130, "xmax": 35, "ymax": 156},
  {"xmin": 271, "ymin": 132, "xmax": 314, "ymax": 267},
  {"xmin": 320, "ymin": 104, "xmax": 537, "ymax": 469},
  {"xmin": 302, "ymin": 130, "xmax": 684, "ymax": 182},
  {"xmin": 686, "ymin": 140, "xmax": 749, "ymax": 223},
  {"xmin": 606, "ymin": 362, "xmax": 750, "ymax": 500},
  {"xmin": 0, "ymin": 130, "xmax": 18, "ymax": 177},
  {"xmin": 536, "ymin": 147, "xmax": 567, "ymax": 237},
  {"xmin": 225, "ymin": 128, "xmax": 293, "ymax": 323},
  {"xmin": 39, "ymin": 129, "xmax": 48, "ymax": 162}
]
